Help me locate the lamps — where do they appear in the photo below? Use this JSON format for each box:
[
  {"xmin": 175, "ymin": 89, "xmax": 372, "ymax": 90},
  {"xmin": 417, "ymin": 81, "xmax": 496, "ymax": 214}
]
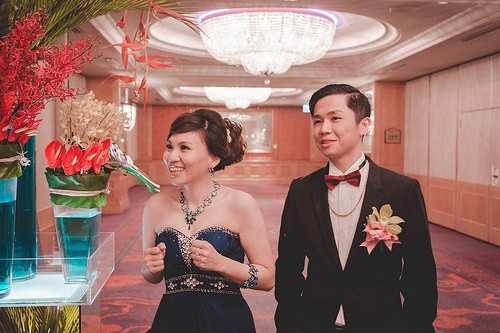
[
  {"xmin": 197, "ymin": 10, "xmax": 340, "ymax": 86},
  {"xmin": 204, "ymin": 87, "xmax": 271, "ymax": 111},
  {"xmin": 120, "ymin": 87, "xmax": 137, "ymax": 132}
]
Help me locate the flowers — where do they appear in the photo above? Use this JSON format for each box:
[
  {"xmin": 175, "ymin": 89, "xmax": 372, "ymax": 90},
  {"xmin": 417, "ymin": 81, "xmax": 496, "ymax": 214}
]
[
  {"xmin": 359, "ymin": 204, "xmax": 404, "ymax": 254},
  {"xmin": 0, "ymin": 0, "xmax": 206, "ymax": 193}
]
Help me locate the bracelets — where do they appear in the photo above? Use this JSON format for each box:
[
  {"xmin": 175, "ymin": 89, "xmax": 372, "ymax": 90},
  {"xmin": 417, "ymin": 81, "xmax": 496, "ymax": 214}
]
[{"xmin": 241, "ymin": 263, "xmax": 259, "ymax": 291}]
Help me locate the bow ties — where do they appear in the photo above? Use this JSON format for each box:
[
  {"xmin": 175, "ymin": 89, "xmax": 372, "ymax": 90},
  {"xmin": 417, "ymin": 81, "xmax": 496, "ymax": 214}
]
[{"xmin": 324, "ymin": 171, "xmax": 361, "ymax": 191}]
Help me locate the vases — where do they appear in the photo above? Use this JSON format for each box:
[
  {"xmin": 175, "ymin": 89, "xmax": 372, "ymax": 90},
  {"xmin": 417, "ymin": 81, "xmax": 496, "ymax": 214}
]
[
  {"xmin": 0, "ymin": 140, "xmax": 21, "ymax": 298},
  {"xmin": 11, "ymin": 138, "xmax": 38, "ymax": 281},
  {"xmin": 45, "ymin": 169, "xmax": 110, "ymax": 283}
]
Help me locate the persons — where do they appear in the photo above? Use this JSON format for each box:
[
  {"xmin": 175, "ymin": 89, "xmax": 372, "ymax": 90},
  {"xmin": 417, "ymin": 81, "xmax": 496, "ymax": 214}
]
[
  {"xmin": 142, "ymin": 109, "xmax": 277, "ymax": 333},
  {"xmin": 272, "ymin": 84, "xmax": 439, "ymax": 333}
]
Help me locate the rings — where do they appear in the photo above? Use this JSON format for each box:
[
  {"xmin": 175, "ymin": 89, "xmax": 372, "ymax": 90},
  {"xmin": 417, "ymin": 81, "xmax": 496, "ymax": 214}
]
[
  {"xmin": 155, "ymin": 261, "xmax": 156, "ymax": 266},
  {"xmin": 196, "ymin": 249, "xmax": 200, "ymax": 255}
]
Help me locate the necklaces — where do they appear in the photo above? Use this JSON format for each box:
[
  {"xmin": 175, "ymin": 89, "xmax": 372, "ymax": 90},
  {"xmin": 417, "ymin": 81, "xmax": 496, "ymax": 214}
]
[
  {"xmin": 328, "ymin": 182, "xmax": 366, "ymax": 217},
  {"xmin": 180, "ymin": 182, "xmax": 221, "ymax": 230}
]
